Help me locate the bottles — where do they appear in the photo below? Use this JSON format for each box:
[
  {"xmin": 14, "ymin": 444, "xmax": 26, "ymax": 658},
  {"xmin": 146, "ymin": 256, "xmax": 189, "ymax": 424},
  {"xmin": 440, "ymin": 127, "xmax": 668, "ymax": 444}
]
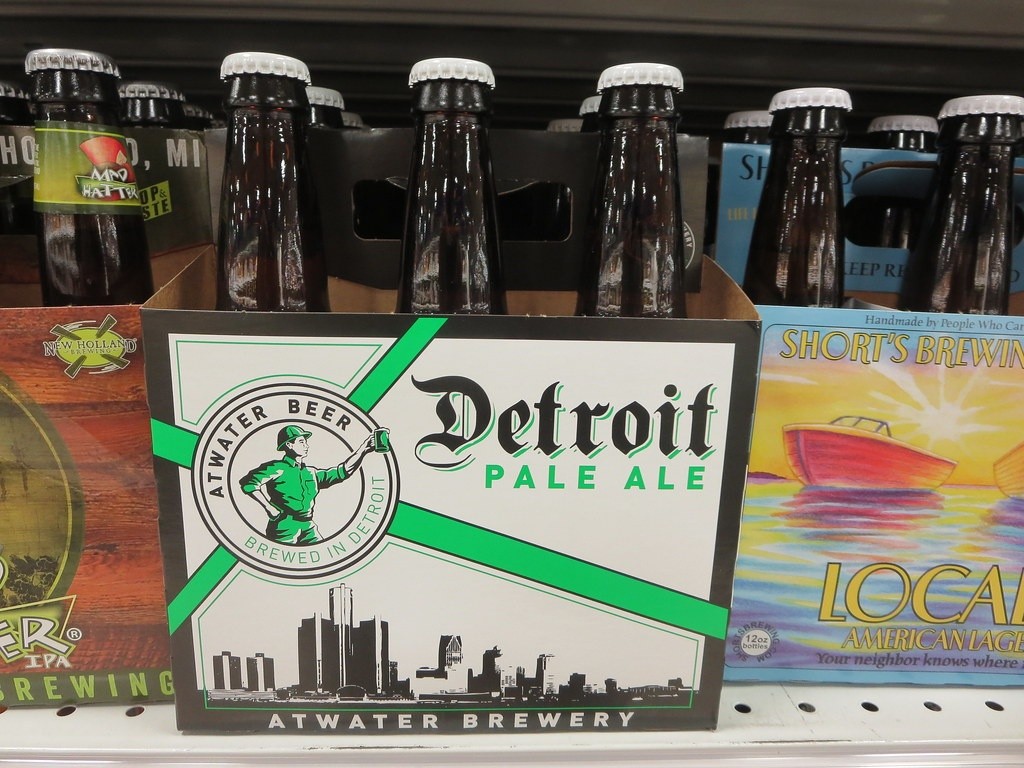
[{"xmin": 0, "ymin": 48, "xmax": 1024, "ymax": 318}]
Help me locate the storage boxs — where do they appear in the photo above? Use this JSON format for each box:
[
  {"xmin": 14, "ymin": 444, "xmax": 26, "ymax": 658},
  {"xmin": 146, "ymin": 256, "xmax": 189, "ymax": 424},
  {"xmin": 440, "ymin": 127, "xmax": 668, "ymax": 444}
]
[{"xmin": 0, "ymin": 125, "xmax": 1024, "ymax": 733}]
